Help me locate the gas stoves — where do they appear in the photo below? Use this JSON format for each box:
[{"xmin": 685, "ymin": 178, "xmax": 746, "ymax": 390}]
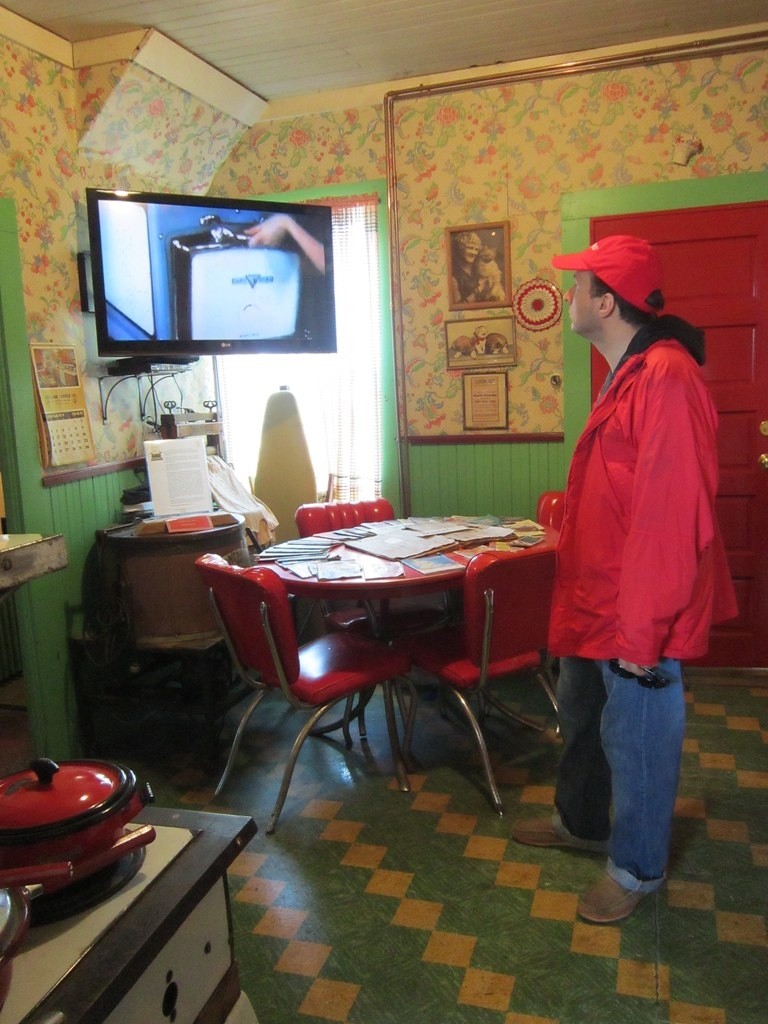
[{"xmin": 0, "ymin": 821, "xmax": 203, "ymax": 1024}]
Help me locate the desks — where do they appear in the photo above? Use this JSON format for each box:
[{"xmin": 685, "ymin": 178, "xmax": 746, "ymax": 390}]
[{"xmin": 255, "ymin": 515, "xmax": 566, "ymax": 795}]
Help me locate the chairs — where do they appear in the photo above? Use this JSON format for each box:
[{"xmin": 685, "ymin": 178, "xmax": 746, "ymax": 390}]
[
  {"xmin": 474, "ymin": 489, "xmax": 579, "ymax": 721},
  {"xmin": 194, "ymin": 553, "xmax": 416, "ymax": 834},
  {"xmin": 401, "ymin": 543, "xmax": 574, "ymax": 817},
  {"xmin": 292, "ymin": 498, "xmax": 452, "ymax": 742}
]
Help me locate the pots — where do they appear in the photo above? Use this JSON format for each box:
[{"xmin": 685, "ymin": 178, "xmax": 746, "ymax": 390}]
[
  {"xmin": 0, "ymin": 822, "xmax": 158, "ymax": 1011},
  {"xmin": 1, "ymin": 756, "xmax": 154, "ymax": 874}
]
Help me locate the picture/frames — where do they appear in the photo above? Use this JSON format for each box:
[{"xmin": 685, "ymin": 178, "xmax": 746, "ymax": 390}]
[
  {"xmin": 462, "ymin": 370, "xmax": 510, "ymax": 431},
  {"xmin": 444, "ymin": 316, "xmax": 518, "ymax": 371},
  {"xmin": 445, "ymin": 220, "xmax": 513, "ymax": 312}
]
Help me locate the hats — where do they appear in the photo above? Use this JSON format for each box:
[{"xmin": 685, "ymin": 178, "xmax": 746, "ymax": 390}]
[{"xmin": 552, "ymin": 235, "xmax": 666, "ymax": 315}]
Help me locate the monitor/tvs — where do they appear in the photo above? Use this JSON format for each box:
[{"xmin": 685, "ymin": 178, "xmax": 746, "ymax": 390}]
[{"xmin": 83, "ymin": 186, "xmax": 338, "ymax": 358}]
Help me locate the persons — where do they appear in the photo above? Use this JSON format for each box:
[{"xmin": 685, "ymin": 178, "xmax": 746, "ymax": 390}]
[
  {"xmin": 508, "ymin": 233, "xmax": 739, "ymax": 925},
  {"xmin": 242, "ymin": 212, "xmax": 326, "ymax": 338}
]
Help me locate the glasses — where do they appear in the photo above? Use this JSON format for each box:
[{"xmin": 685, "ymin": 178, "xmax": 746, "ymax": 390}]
[{"xmin": 608, "ymin": 658, "xmax": 670, "ymax": 689}]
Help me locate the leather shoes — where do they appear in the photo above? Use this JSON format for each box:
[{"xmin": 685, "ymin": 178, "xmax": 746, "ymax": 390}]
[
  {"xmin": 576, "ymin": 871, "xmax": 650, "ymax": 922},
  {"xmin": 511, "ymin": 818, "xmax": 595, "ymax": 852}
]
